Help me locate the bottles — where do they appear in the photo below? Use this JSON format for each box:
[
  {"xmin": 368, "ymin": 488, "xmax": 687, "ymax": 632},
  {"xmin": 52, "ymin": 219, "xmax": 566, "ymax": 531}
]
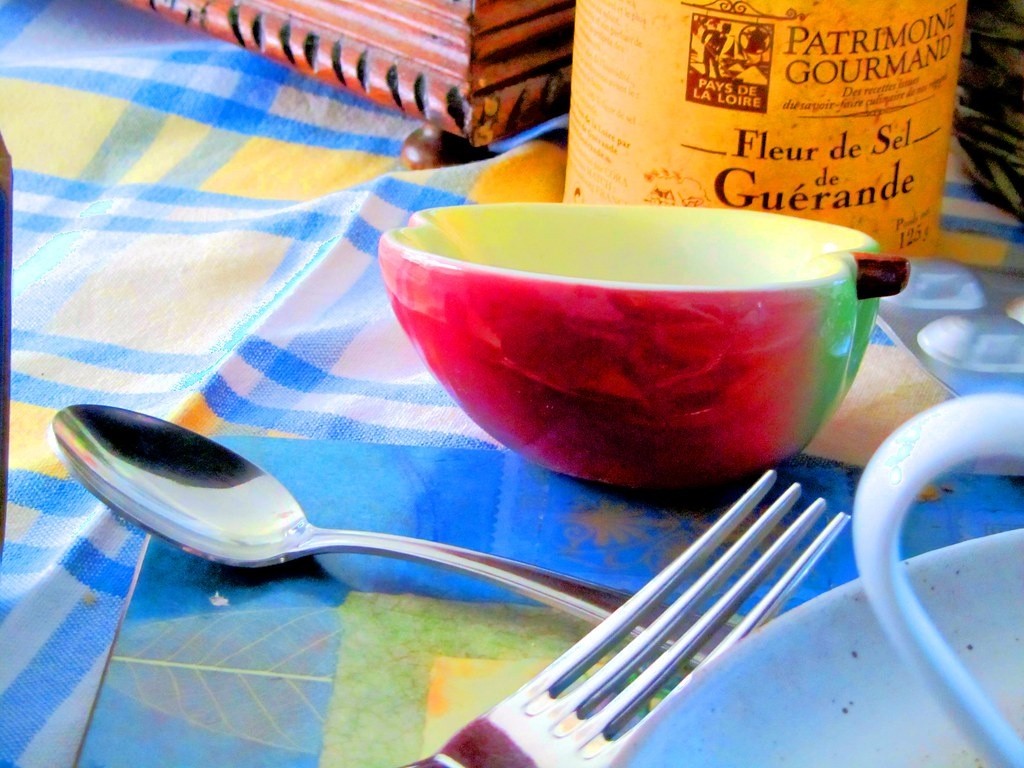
[{"xmin": 560, "ymin": 0, "xmax": 972, "ymax": 262}]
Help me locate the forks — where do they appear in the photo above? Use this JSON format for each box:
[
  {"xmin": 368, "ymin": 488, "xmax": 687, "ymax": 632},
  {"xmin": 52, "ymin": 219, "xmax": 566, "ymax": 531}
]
[{"xmin": 381, "ymin": 467, "xmax": 852, "ymax": 768}]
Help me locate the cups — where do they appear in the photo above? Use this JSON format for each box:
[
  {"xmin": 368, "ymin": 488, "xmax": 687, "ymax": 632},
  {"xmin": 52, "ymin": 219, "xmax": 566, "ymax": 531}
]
[{"xmin": 847, "ymin": 391, "xmax": 1024, "ymax": 768}]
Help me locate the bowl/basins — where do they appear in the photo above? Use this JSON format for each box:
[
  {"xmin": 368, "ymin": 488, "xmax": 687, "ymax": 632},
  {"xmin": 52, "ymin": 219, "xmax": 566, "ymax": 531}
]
[{"xmin": 376, "ymin": 203, "xmax": 909, "ymax": 487}]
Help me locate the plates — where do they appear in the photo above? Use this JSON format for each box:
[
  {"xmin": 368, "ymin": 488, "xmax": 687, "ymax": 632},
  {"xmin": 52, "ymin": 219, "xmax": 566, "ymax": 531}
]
[{"xmin": 610, "ymin": 526, "xmax": 1024, "ymax": 768}]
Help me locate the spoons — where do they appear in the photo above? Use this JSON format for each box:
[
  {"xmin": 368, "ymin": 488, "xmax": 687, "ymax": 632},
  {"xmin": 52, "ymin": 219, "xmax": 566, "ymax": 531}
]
[{"xmin": 51, "ymin": 404, "xmax": 771, "ymax": 666}]
